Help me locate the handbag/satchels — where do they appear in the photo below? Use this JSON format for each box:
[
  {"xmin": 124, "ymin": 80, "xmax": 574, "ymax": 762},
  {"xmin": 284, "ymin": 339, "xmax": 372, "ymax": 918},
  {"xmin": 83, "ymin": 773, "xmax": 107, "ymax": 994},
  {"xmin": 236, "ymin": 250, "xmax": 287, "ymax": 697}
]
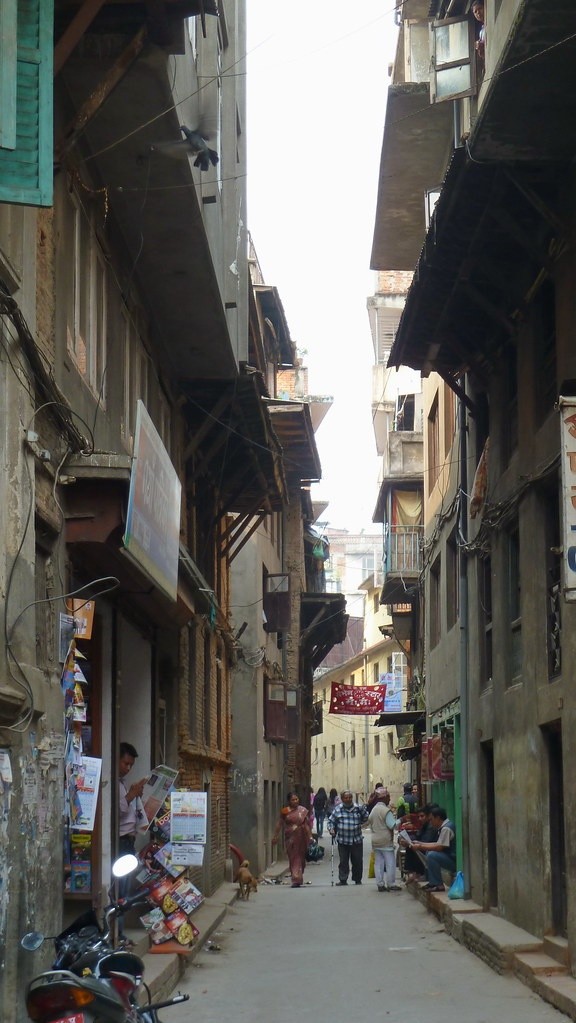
[
  {"xmin": 447, "ymin": 871, "xmax": 464, "ymax": 899},
  {"xmin": 304, "ymin": 840, "xmax": 325, "ymax": 863},
  {"xmin": 367, "ymin": 852, "xmax": 376, "ymax": 878}
]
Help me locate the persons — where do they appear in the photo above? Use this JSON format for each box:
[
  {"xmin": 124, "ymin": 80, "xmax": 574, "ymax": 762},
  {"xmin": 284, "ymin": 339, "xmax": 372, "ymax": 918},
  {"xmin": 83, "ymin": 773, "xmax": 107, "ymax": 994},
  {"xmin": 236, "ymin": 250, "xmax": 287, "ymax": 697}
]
[
  {"xmin": 368, "ymin": 787, "xmax": 408, "ymax": 892},
  {"xmin": 313, "ymin": 788, "xmax": 328, "ymax": 838},
  {"xmin": 273, "ymin": 790, "xmax": 315, "ymax": 888},
  {"xmin": 327, "ymin": 789, "xmax": 369, "ymax": 886},
  {"xmin": 368, "ymin": 783, "xmax": 383, "ymax": 803},
  {"xmin": 396, "ymin": 783, "xmax": 457, "ymax": 892},
  {"xmin": 118, "ymin": 742, "xmax": 149, "ymax": 946},
  {"xmin": 310, "ymin": 789, "xmax": 316, "ymax": 831},
  {"xmin": 325, "ymin": 788, "xmax": 341, "ymax": 819}
]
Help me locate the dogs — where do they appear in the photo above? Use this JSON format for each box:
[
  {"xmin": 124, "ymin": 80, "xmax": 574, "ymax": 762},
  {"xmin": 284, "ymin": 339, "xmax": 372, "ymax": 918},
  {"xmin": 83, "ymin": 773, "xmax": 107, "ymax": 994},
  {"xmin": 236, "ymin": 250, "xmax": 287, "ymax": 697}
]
[{"xmin": 233, "ymin": 860, "xmax": 257, "ymax": 901}]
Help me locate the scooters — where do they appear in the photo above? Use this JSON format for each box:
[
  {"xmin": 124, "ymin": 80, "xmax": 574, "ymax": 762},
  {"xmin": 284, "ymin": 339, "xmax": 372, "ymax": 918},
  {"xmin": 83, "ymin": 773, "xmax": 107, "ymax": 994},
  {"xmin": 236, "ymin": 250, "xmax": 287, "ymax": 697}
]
[{"xmin": 21, "ymin": 852, "xmax": 190, "ymax": 1023}]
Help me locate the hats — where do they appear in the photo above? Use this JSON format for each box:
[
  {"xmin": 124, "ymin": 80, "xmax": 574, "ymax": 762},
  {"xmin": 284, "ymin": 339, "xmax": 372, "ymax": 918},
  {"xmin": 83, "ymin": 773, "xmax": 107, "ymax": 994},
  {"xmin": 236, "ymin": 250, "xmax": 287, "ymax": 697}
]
[{"xmin": 402, "ymin": 783, "xmax": 413, "ymax": 790}]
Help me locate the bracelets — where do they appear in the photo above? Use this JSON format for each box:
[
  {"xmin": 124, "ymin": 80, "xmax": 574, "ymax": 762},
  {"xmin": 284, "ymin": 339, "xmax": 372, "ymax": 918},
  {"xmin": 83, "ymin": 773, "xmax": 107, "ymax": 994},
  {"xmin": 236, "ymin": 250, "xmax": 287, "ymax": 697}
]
[{"xmin": 309, "ymin": 837, "xmax": 313, "ymax": 840}]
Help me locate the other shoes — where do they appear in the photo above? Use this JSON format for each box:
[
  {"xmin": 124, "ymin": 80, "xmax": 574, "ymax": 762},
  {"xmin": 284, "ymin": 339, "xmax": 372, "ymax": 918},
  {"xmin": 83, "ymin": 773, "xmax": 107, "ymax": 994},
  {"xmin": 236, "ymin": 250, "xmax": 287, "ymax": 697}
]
[
  {"xmin": 378, "ymin": 886, "xmax": 389, "ymax": 892},
  {"xmin": 336, "ymin": 880, "xmax": 348, "ymax": 886},
  {"xmin": 291, "ymin": 883, "xmax": 300, "ymax": 888},
  {"xmin": 387, "ymin": 885, "xmax": 402, "ymax": 891},
  {"xmin": 422, "ymin": 883, "xmax": 430, "ymax": 889},
  {"xmin": 426, "ymin": 884, "xmax": 445, "ymax": 892},
  {"xmin": 408, "ymin": 873, "xmax": 421, "ymax": 880},
  {"xmin": 356, "ymin": 881, "xmax": 362, "ymax": 884},
  {"xmin": 414, "ymin": 875, "xmax": 427, "ymax": 881}
]
